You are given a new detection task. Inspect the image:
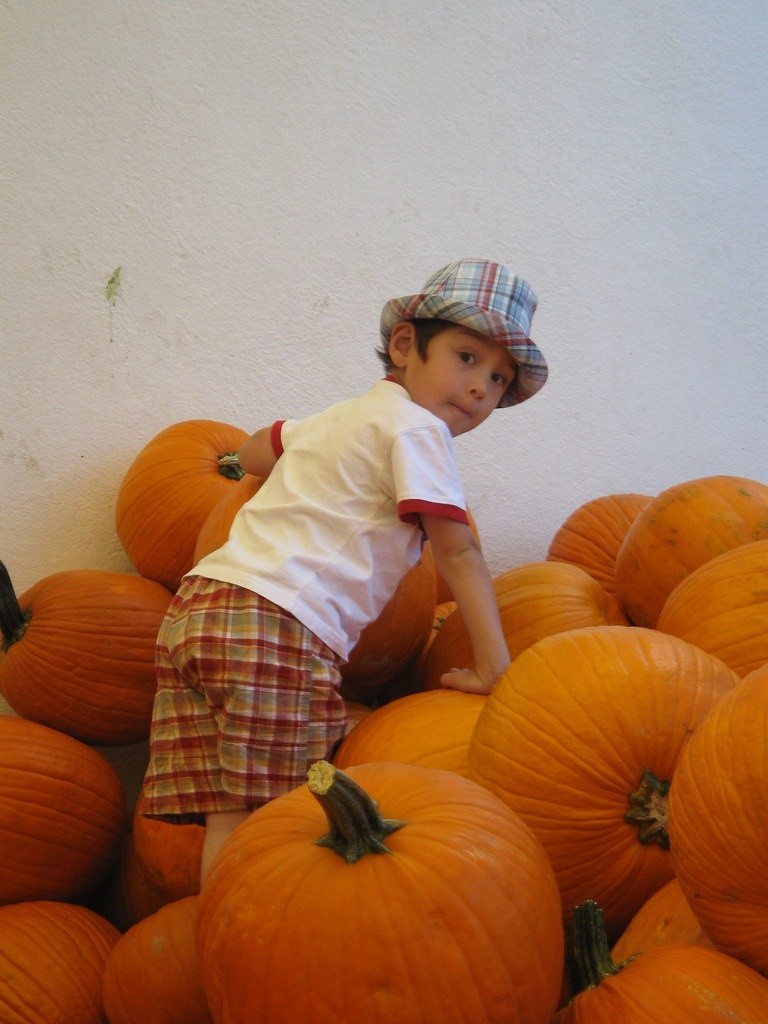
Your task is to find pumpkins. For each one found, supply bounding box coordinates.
[0,418,768,1024]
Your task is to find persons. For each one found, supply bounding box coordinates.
[140,258,549,893]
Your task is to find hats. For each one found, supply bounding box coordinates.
[380,257,548,410]
